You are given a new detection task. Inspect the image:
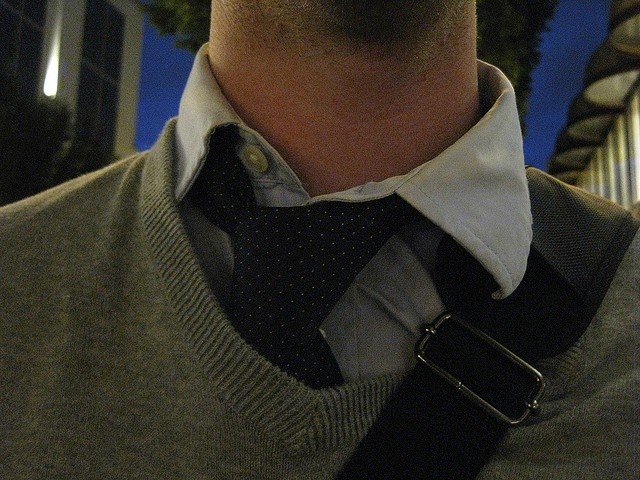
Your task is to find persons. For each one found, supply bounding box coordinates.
[0,0,640,480]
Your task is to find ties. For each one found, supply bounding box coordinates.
[187,138,418,389]
